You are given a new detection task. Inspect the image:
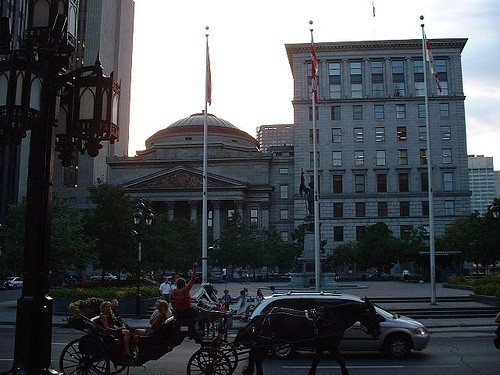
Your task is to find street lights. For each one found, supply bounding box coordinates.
[129,199,157,314]
[0,13,122,374]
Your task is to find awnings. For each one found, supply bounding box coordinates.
[419,251,462,255]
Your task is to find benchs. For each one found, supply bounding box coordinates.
[141,316,175,338]
[86,315,120,343]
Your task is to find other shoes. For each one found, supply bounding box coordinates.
[123,353,135,360]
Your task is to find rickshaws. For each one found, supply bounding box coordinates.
[60,296,240,375]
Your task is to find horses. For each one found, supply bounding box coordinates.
[233,295,382,375]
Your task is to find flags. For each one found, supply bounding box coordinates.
[425,38,442,93]
[311,42,319,103]
[207,41,211,104]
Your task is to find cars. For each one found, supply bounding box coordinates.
[89,272,117,281]
[244,291,431,357]
[2,276,23,289]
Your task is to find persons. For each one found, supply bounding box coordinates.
[299,168,308,197]
[255,288,264,302]
[236,286,252,302]
[308,181,313,215]
[130,299,168,351]
[270,286,278,294]
[214,289,231,311]
[238,265,250,284]
[159,275,180,303]
[100,297,133,356]
[403,267,410,284]
[170,262,206,336]
[375,263,382,281]
